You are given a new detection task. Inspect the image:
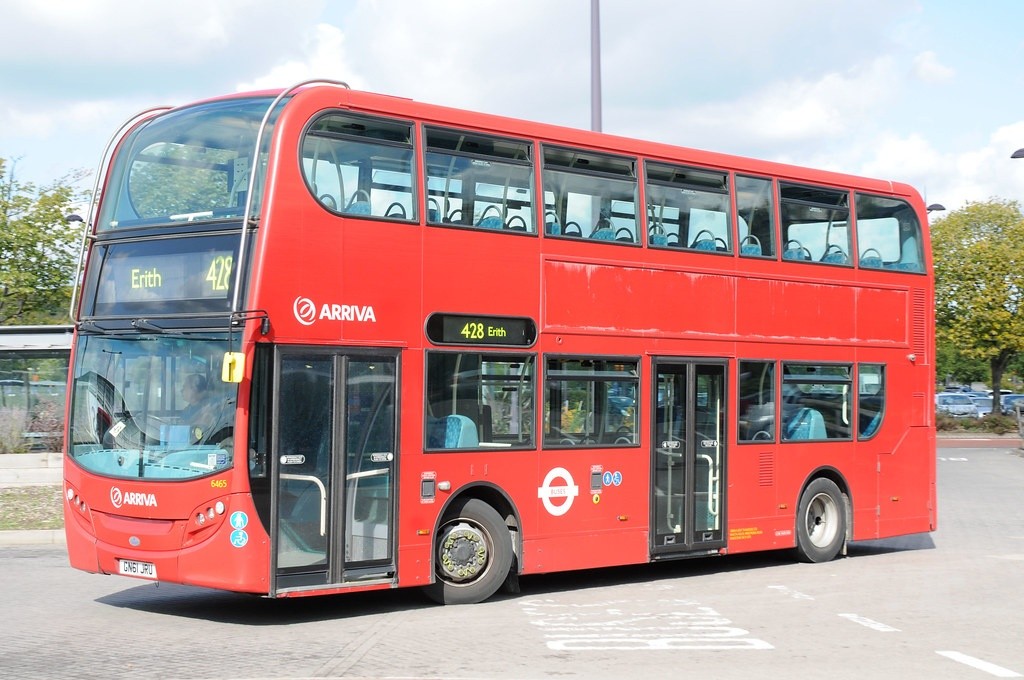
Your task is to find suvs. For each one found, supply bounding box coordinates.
[944,385,975,394]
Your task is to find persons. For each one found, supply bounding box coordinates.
[166,374,214,430]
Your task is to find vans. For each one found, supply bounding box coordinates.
[859,373,884,395]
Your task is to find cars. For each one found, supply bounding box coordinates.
[971,397,995,417]
[606,395,637,417]
[981,390,1012,397]
[999,394,1024,414]
[936,392,979,420]
[965,392,989,398]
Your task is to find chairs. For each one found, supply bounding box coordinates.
[448,209,468,225]
[819,244,845,264]
[689,229,716,251]
[860,247,883,268]
[429,197,442,222]
[343,187,373,215]
[474,205,503,228]
[546,211,561,235]
[506,215,527,231]
[741,235,762,255]
[835,250,848,260]
[563,221,582,237]
[383,201,407,218]
[716,237,728,251]
[649,224,669,246]
[616,227,634,241]
[588,217,616,240]
[783,238,805,260]
[357,378,883,522]
[319,192,338,209]
[799,246,812,260]
[667,231,682,246]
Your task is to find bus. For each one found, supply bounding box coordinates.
[72,79,941,602]
